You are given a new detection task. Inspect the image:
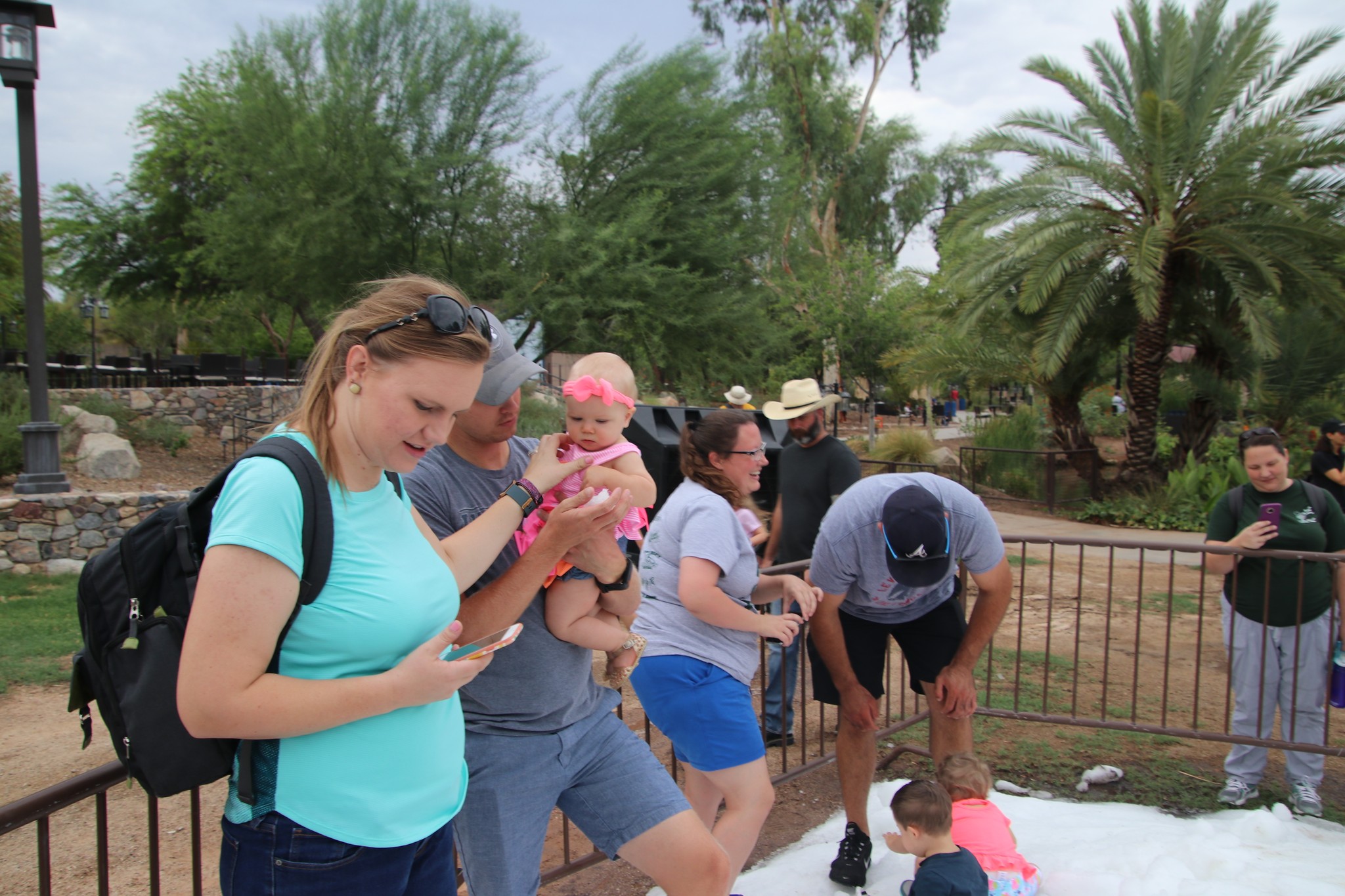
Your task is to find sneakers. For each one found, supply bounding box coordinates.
[1217,777,1259,805]
[1288,784,1323,817]
[829,821,872,888]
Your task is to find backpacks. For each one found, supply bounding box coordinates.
[66,435,335,799]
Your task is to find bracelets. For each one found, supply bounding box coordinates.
[516,478,544,505]
[595,557,632,593]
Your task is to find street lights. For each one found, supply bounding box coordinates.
[820,380,851,436]
[80,290,111,385]
[0,0,70,496]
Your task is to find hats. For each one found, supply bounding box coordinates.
[723,385,753,405]
[881,484,951,589]
[762,378,843,421]
[460,305,550,406]
[1321,418,1345,435]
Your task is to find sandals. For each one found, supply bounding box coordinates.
[603,632,647,691]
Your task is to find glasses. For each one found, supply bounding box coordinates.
[363,294,493,346]
[718,442,767,461]
[1238,426,1280,442]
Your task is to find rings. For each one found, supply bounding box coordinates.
[529,449,537,458]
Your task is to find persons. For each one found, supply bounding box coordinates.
[1309,420,1344,513]
[804,471,1016,887]
[838,385,959,426]
[881,747,1043,895]
[1202,427,1345,816]
[888,778,989,896]
[173,275,597,895]
[752,376,862,746]
[732,490,770,549]
[1112,390,1127,416]
[719,385,756,409]
[388,308,738,895]
[513,353,657,688]
[625,410,823,879]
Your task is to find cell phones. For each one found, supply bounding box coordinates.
[766,612,803,644]
[439,623,524,660]
[1260,503,1281,539]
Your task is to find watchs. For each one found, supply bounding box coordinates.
[500,480,537,517]
